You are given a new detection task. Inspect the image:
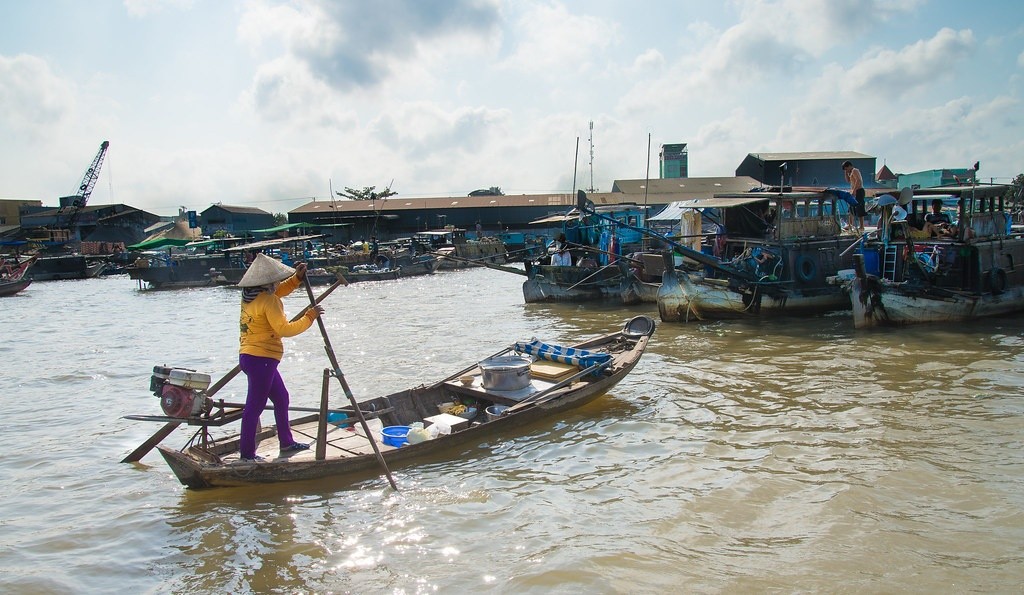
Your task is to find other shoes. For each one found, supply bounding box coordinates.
[280,442,309,451]
[242,455,266,461]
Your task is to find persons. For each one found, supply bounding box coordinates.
[842,160,865,231]
[246,249,261,263]
[924,199,950,228]
[411,237,420,251]
[370,237,378,254]
[783,201,793,218]
[476,222,481,239]
[306,240,312,251]
[428,234,441,243]
[238,253,325,461]
[361,239,368,253]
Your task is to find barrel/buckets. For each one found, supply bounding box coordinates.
[381,425,413,448]
[853,239,881,277]
[438,402,454,413]
[485,405,511,422]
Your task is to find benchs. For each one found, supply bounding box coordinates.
[445,375,557,406]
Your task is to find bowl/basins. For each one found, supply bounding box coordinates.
[459,375,475,386]
[459,408,478,419]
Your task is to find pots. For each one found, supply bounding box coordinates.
[478,355,531,391]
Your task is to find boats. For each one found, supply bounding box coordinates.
[0,131,1024,334]
[123,311,658,492]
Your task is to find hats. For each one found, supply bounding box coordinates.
[238,253,296,287]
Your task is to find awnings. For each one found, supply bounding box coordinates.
[646,200,705,221]
[679,197,770,208]
[528,215,586,224]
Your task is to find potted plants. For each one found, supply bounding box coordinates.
[214,229,227,239]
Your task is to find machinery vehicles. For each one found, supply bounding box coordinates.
[20,139,110,257]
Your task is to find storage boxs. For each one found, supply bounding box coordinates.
[530,359,581,383]
[423,412,469,438]
[459,407,477,419]
[963,211,1012,238]
[169,370,212,390]
[137,259,150,269]
[152,365,172,379]
[898,168,976,189]
[838,268,857,281]
[353,418,384,443]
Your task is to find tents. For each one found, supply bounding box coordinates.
[264,221,356,237]
[127,238,222,256]
